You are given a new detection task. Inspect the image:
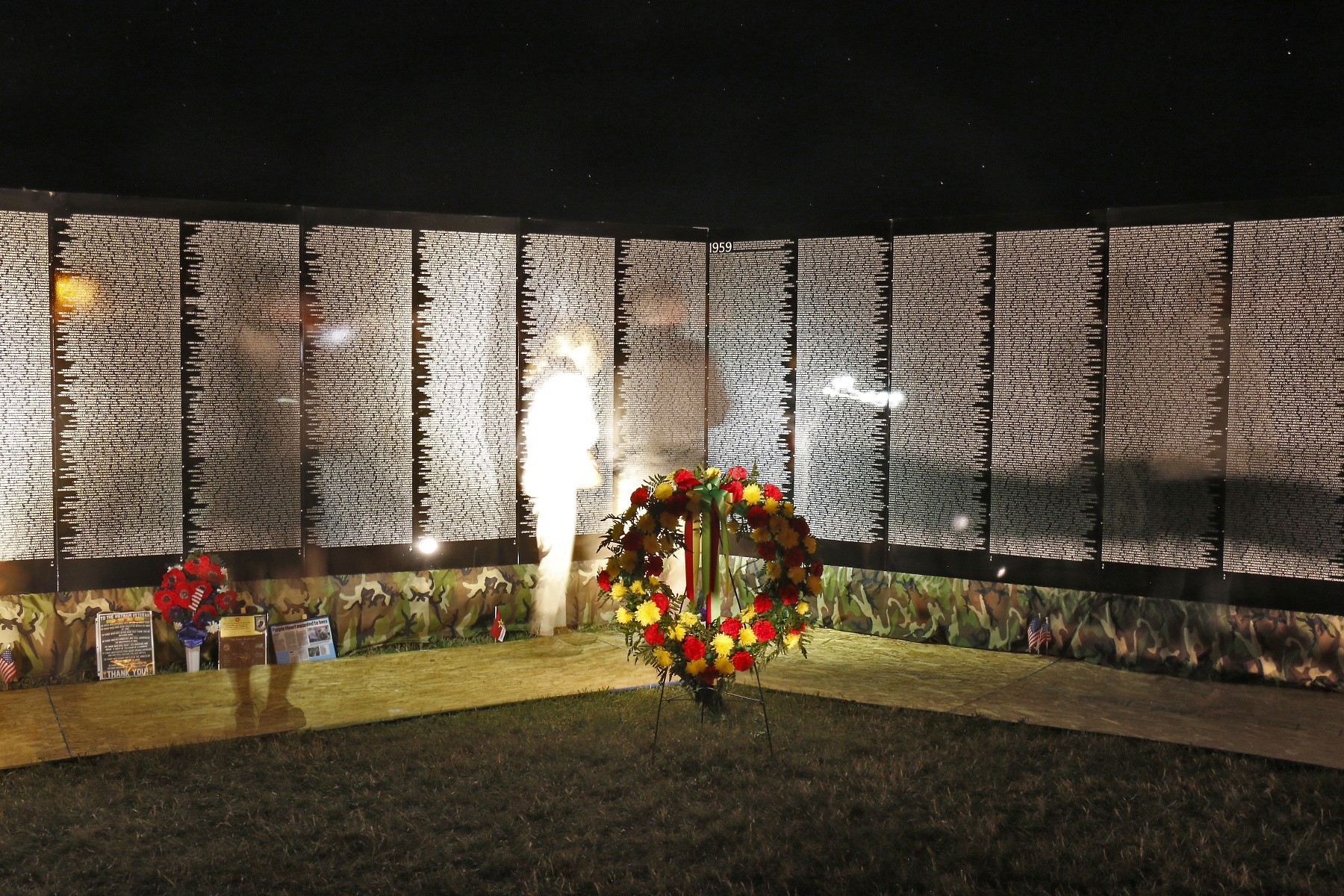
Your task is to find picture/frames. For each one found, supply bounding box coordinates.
[267,614,339,663]
[215,613,270,669]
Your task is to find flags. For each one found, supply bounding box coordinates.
[1027,616,1052,653]
[489,609,506,642]
[0,647,18,686]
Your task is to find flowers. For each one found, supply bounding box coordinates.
[593,465,824,692]
[153,549,238,637]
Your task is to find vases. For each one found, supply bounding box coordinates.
[181,638,202,673]
